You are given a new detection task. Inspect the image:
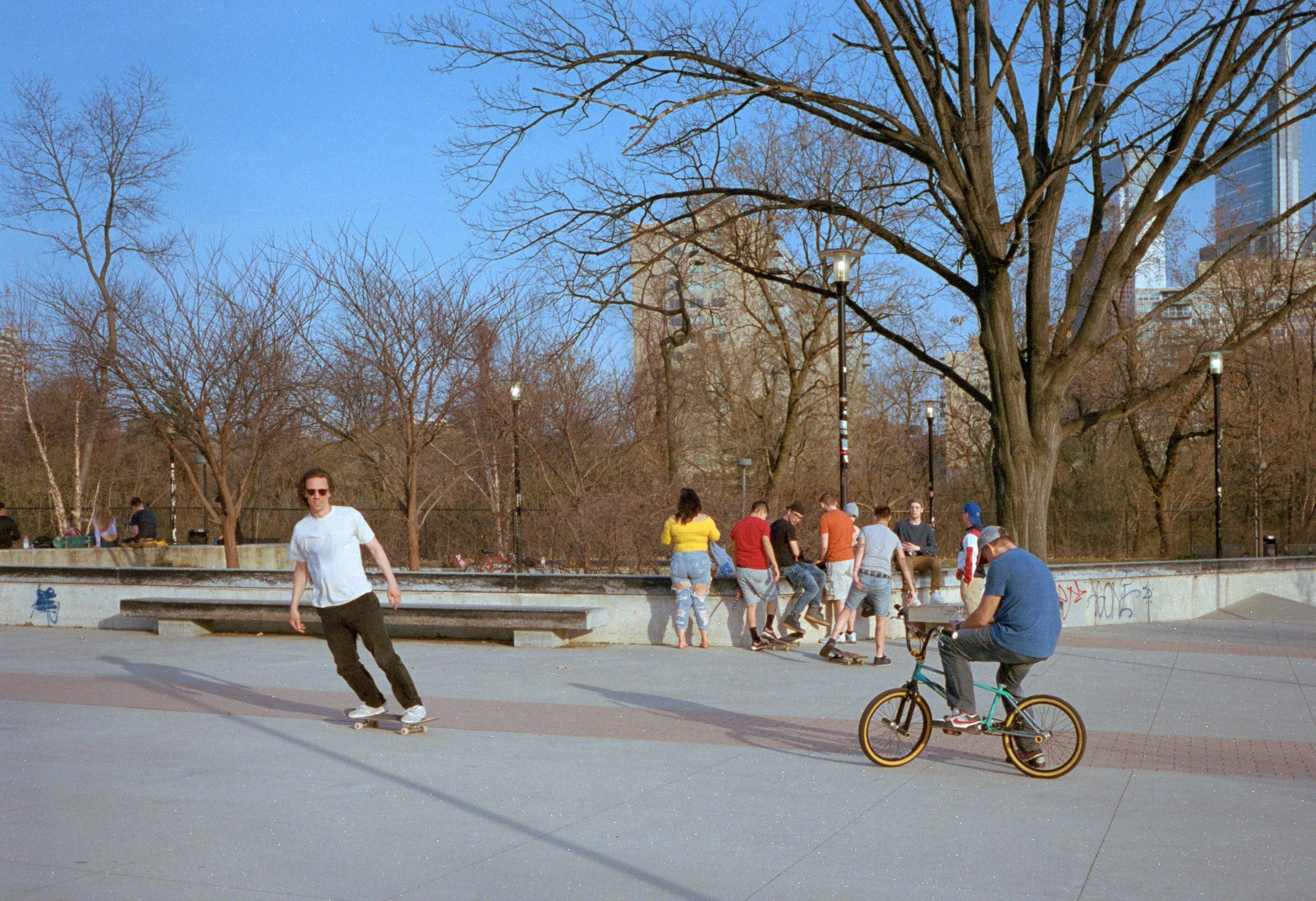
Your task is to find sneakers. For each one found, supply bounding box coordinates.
[844,632,857,643]
[907,590,921,607]
[929,589,948,605]
[805,606,830,626]
[782,615,806,634]
[1005,748,1046,768]
[873,653,892,666]
[400,705,426,723]
[760,627,778,641]
[819,638,836,658]
[347,700,389,719]
[819,632,846,643]
[860,605,876,617]
[929,710,982,735]
[750,636,768,650]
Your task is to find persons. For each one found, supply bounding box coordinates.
[770,501,829,635]
[0,502,20,549]
[956,502,986,616]
[938,526,1062,769]
[661,489,720,648]
[93,503,120,548]
[121,497,157,543]
[893,500,948,607]
[288,468,426,724]
[819,505,915,666]
[812,488,859,643]
[843,502,860,536]
[730,501,779,652]
[214,496,242,545]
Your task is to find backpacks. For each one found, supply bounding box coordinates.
[65,526,83,536]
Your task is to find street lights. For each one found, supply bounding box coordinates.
[158,414,182,545]
[1200,349,1235,560]
[917,400,943,531]
[818,247,867,516]
[737,457,752,519]
[502,379,530,574]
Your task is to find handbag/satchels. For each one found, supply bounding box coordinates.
[710,541,736,578]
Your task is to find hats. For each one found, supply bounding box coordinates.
[963,502,982,525]
[842,502,859,519]
[786,501,804,516]
[910,498,926,514]
[976,526,1009,566]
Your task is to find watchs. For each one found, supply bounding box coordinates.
[956,623,961,630]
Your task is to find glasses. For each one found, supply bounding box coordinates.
[790,510,803,521]
[304,489,329,497]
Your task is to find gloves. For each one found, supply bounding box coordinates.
[214,536,219,545]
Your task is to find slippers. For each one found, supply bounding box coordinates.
[699,643,710,648]
[678,644,688,649]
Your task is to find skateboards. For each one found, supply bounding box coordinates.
[344,708,440,735]
[827,647,868,665]
[53,536,95,548]
[769,633,803,651]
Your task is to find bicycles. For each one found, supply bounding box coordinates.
[462,548,531,575]
[858,603,1088,779]
